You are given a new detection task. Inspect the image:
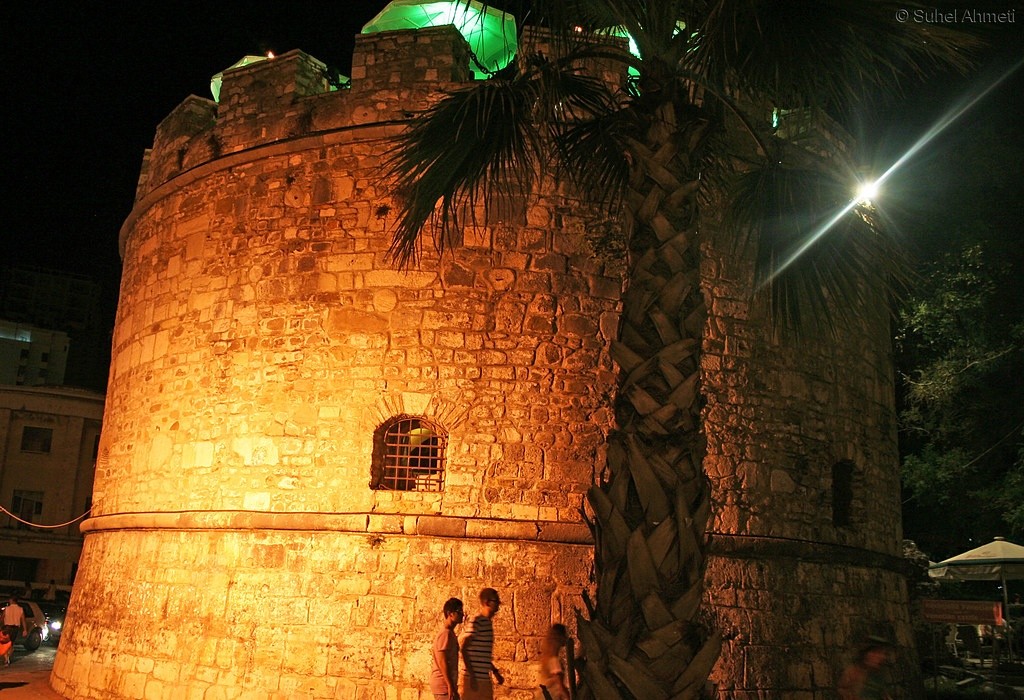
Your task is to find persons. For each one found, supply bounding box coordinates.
[540,623,570,700]
[992,618,1013,638]
[19,583,32,599]
[0,597,28,667]
[459,589,504,700]
[43,579,56,600]
[430,598,461,700]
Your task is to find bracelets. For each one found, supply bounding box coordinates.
[493,669,498,674]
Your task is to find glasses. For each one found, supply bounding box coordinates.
[489,599,500,604]
[451,610,463,614]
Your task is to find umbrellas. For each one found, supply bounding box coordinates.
[211,53,349,102]
[928,536,1024,663]
[361,0,520,80]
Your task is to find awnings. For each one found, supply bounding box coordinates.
[0,540,82,562]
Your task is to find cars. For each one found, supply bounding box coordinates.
[0,599,66,651]
